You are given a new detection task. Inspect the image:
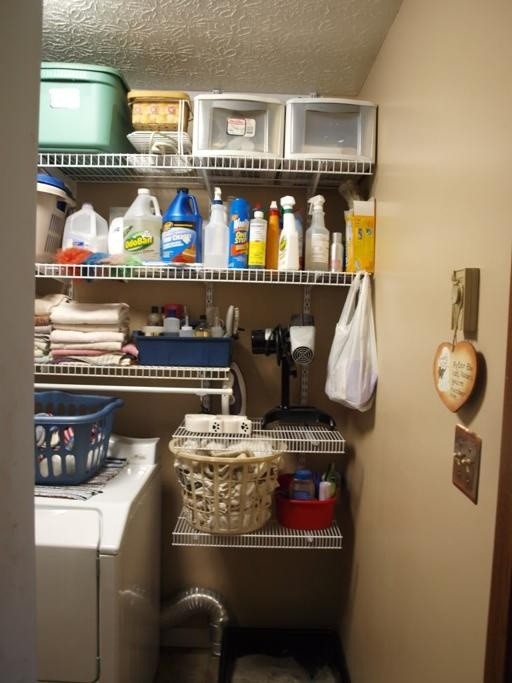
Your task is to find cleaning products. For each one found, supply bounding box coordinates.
[59,185,346,273]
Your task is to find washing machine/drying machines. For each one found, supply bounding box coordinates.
[32,432,170,683]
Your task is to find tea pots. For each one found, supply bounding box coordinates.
[211,307,225,337]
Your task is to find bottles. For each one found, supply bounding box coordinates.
[194,315,211,337]
[163,308,180,336]
[180,313,194,336]
[320,460,342,488]
[330,231,344,272]
[229,196,250,268]
[147,305,164,326]
[289,469,316,500]
[249,202,268,268]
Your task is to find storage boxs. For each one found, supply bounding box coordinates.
[38,62,142,181]
[192,94,285,185]
[132,331,232,367]
[277,98,377,189]
[36,173,77,264]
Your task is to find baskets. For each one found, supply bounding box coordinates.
[34,391,124,486]
[169,432,287,535]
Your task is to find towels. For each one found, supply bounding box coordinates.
[34,294,139,367]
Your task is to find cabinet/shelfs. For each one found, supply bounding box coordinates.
[34,153,374,402]
[171,417,348,549]
